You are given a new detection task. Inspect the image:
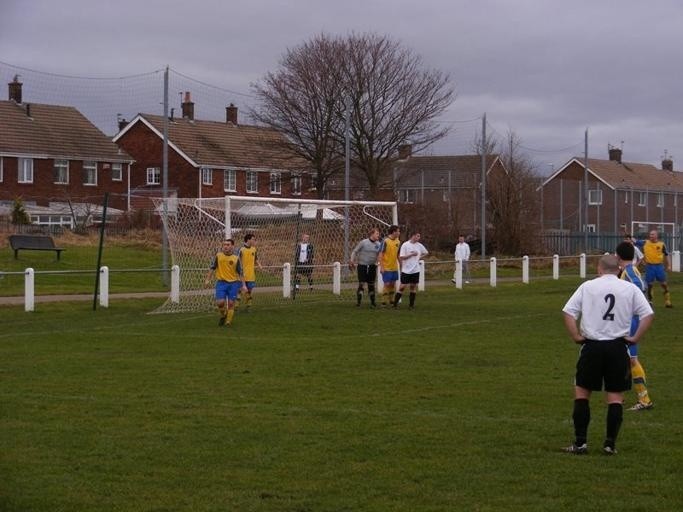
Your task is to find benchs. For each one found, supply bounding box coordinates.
[8,234,67,261]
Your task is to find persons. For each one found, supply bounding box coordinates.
[347,227,381,309]
[379,223,401,307]
[616,233,644,280]
[203,239,248,327]
[292,233,315,295]
[392,229,429,309]
[634,228,672,309]
[450,234,473,284]
[607,241,655,414]
[234,234,263,308]
[558,253,655,456]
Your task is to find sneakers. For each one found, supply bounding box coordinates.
[218,316,226,326]
[604,446,617,454]
[562,442,588,453]
[627,401,653,410]
